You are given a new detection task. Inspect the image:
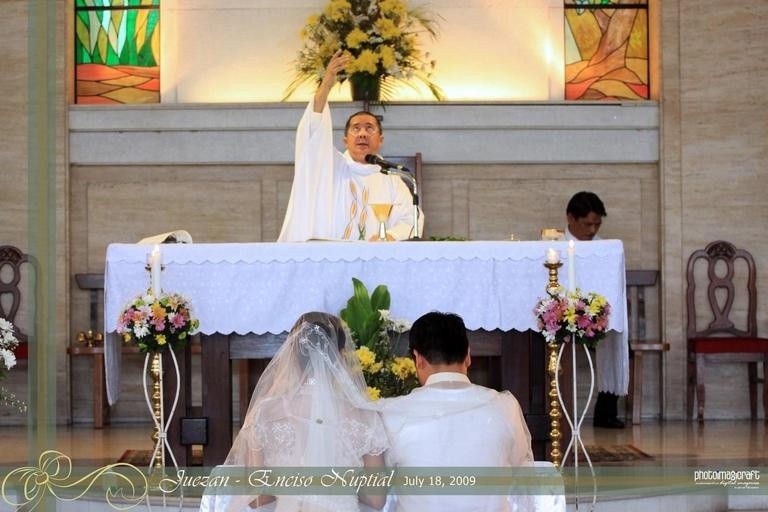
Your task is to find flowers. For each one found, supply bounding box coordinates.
[114,287,201,355]
[279,0,448,112]
[531,288,612,348]
[340,276,422,400]
[0,317,28,416]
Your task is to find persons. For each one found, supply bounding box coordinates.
[272,49,428,244]
[198,310,392,511]
[556,189,629,430]
[378,311,538,511]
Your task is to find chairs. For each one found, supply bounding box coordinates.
[0,244,44,431]
[683,239,768,424]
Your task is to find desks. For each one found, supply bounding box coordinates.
[103,238,628,461]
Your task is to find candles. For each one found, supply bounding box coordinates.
[547,251,560,264]
[567,246,576,295]
[150,249,162,297]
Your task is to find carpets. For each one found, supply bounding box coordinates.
[115,442,658,467]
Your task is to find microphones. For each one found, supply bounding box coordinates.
[365,154,410,172]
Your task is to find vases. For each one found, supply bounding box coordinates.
[348,76,382,104]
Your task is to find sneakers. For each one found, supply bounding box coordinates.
[593,416,626,428]
